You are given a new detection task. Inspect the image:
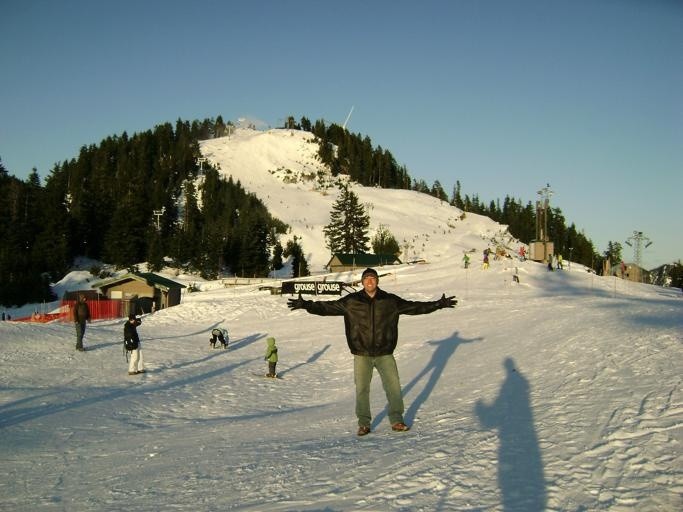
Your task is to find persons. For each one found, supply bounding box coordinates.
[495,245,501,258]
[1,311,5,321]
[6,314,10,321]
[264,336,279,378]
[461,254,469,269]
[123,313,148,375]
[73,294,91,351]
[556,252,563,270]
[546,253,553,272]
[482,247,495,257]
[286,268,458,436]
[209,328,229,349]
[511,267,519,283]
[482,254,489,270]
[519,246,527,261]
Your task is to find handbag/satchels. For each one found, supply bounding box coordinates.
[123,336,138,351]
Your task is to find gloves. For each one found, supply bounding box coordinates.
[438,292,458,309]
[286,292,308,312]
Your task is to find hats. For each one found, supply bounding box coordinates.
[361,267,378,280]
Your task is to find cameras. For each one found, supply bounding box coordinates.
[138,320,141,324]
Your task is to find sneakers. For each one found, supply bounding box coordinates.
[357,427,371,436]
[391,422,409,432]
[128,370,145,375]
[266,373,279,378]
[76,346,86,351]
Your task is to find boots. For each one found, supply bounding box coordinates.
[210,342,227,350]
